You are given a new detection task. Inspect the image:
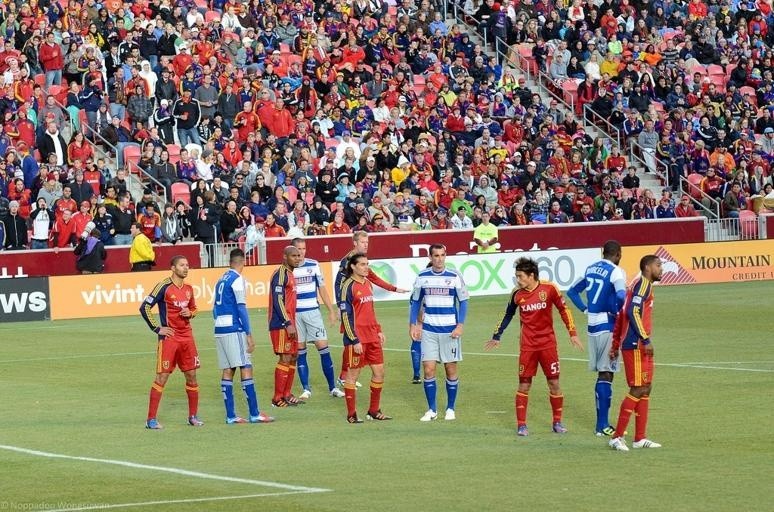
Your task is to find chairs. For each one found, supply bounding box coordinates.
[0,0,772,249]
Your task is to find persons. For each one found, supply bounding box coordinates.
[434,1,774,238]
[0,1,701,274]
[290,237,346,399]
[139,255,205,430]
[608,255,663,452]
[334,230,412,390]
[482,257,584,436]
[339,252,393,423]
[566,240,629,436]
[409,261,433,384]
[409,244,469,421]
[212,249,275,424]
[268,245,306,408]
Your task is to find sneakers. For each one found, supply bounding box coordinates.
[596,425,628,436]
[366,410,392,420]
[632,438,662,449]
[413,376,421,383]
[271,391,311,407]
[420,409,437,421]
[146,420,161,429]
[225,411,275,424]
[552,423,567,433]
[346,412,363,423]
[330,377,361,397]
[517,426,528,435]
[187,417,204,427]
[445,408,455,420]
[609,438,630,452]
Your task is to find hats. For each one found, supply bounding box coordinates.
[630,108,636,113]
[503,152,537,170]
[366,155,376,163]
[495,136,503,141]
[417,133,429,142]
[550,100,557,105]
[764,127,773,134]
[9,201,19,210]
